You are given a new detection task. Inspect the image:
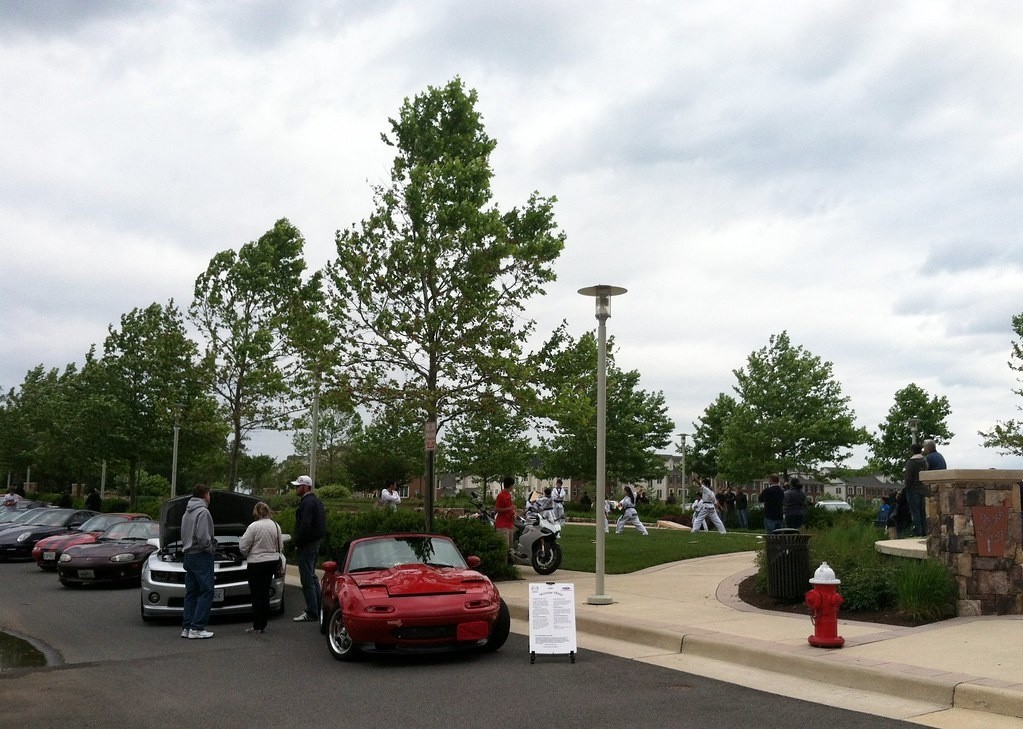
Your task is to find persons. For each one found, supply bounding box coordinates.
[580,491,592,507]
[472,508,495,526]
[84,488,103,512]
[459,509,471,518]
[758,473,806,534]
[690,475,726,533]
[526,478,566,539]
[15,480,25,498]
[291,475,326,622]
[434,508,453,520]
[382,479,401,512]
[239,502,284,634]
[3,486,18,507]
[716,486,749,528]
[604,493,611,532]
[494,476,517,566]
[59,488,73,508]
[281,489,287,495]
[181,483,218,638]
[877,439,946,539]
[373,492,376,498]
[616,486,649,536]
[667,492,677,504]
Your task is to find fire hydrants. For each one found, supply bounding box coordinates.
[804,561,846,649]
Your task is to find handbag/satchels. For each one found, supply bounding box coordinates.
[277,566,285,577]
[377,499,390,508]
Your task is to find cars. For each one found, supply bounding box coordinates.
[31,511,161,573]
[814,500,854,513]
[137,487,292,624]
[55,520,161,589]
[0,492,105,560]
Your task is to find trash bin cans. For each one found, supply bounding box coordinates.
[762,529,813,607]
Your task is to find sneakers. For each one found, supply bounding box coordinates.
[181,628,189,638]
[188,629,214,639]
[293,612,316,621]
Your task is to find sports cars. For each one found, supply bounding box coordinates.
[317,531,512,663]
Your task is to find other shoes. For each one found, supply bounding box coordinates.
[246,627,262,633]
[906,534,922,538]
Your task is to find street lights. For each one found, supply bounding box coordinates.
[676,433,691,514]
[576,284,629,606]
[170,402,189,498]
[301,365,328,494]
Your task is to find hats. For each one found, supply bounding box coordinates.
[291,476,312,486]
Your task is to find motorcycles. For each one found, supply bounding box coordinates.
[467,489,562,575]
